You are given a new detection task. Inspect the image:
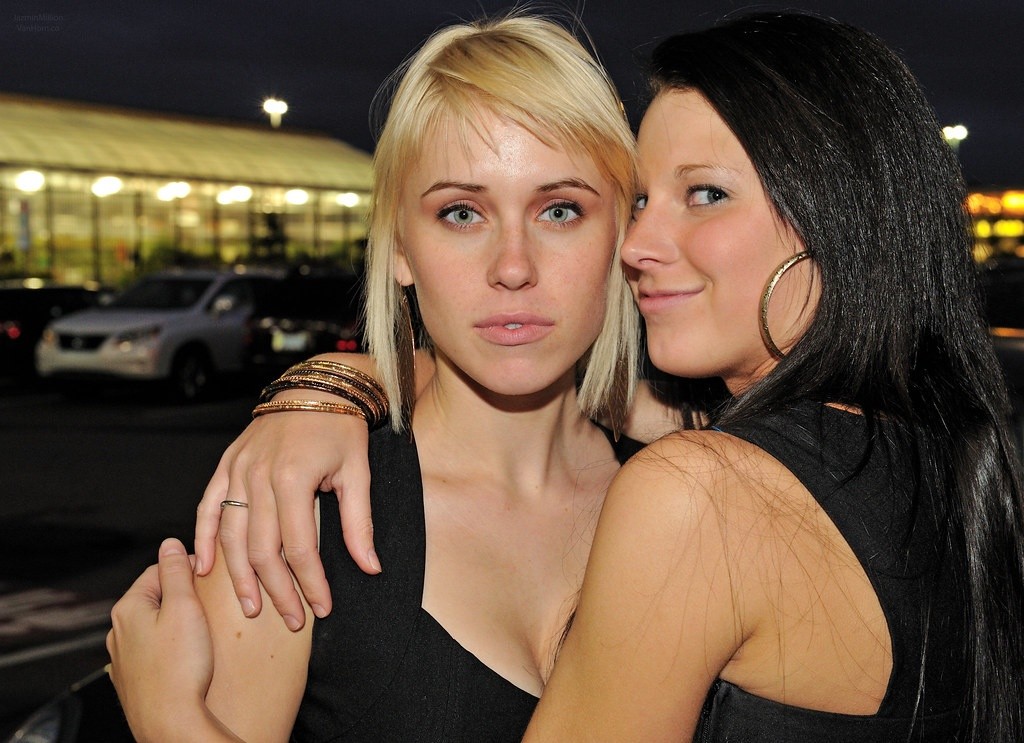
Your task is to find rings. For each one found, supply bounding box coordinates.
[220,499,250,511]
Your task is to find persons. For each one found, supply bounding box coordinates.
[75,8,726,743]
[104,3,1024,743]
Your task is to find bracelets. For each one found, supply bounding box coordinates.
[251,397,363,427]
[265,358,392,424]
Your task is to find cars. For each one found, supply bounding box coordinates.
[244,261,383,398]
[0,282,109,381]
[34,265,280,404]
